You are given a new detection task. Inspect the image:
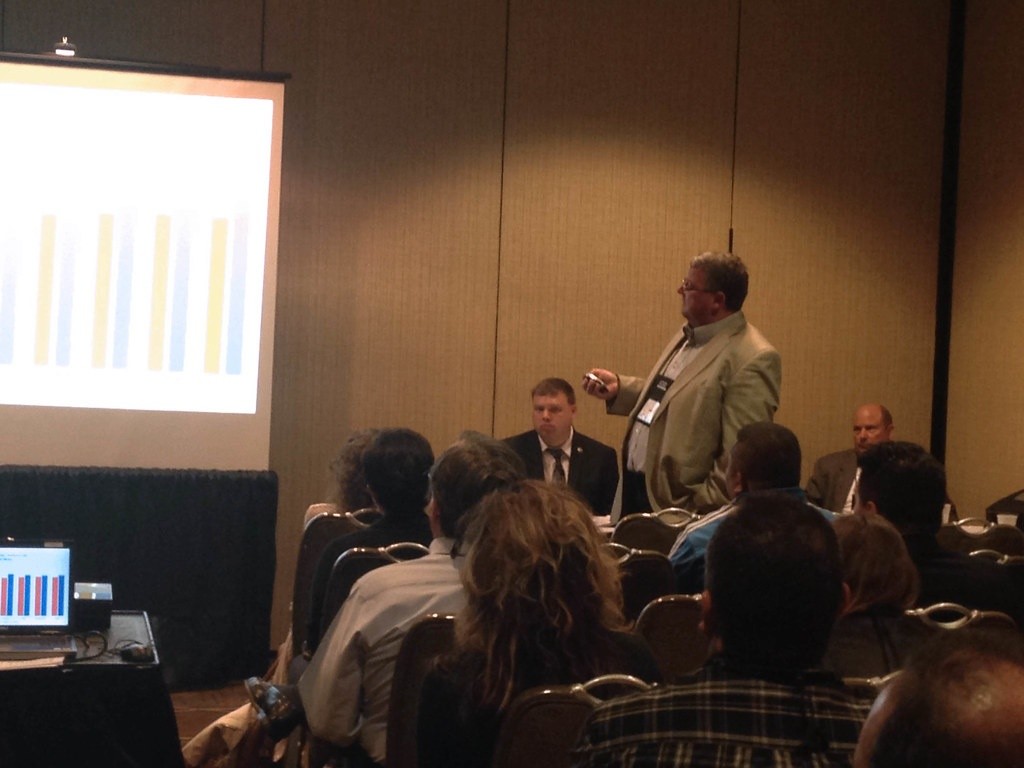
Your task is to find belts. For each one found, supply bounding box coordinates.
[622,471,646,486]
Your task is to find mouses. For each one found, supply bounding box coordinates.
[120,642,152,662]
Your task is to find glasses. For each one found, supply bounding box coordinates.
[681,278,716,296]
[328,455,340,470]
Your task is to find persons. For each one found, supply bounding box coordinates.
[415,478,665,767]
[304,429,435,653]
[833,512,939,677]
[668,420,838,595]
[853,627,1024,768]
[502,378,620,515]
[305,428,380,526]
[806,404,895,513]
[580,250,783,521]
[852,441,1024,623]
[296,428,528,768]
[565,495,871,768]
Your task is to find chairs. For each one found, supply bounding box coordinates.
[294,508,1024,768]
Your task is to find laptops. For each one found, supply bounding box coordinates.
[0,539,77,660]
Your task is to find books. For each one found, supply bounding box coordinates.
[0,635,77,661]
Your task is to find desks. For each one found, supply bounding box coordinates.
[0,608,187,768]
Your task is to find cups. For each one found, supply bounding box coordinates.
[996,514,1018,527]
[942,504,952,524]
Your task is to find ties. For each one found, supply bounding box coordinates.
[546,449,567,489]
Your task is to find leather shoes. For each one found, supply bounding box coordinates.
[244,676,305,743]
